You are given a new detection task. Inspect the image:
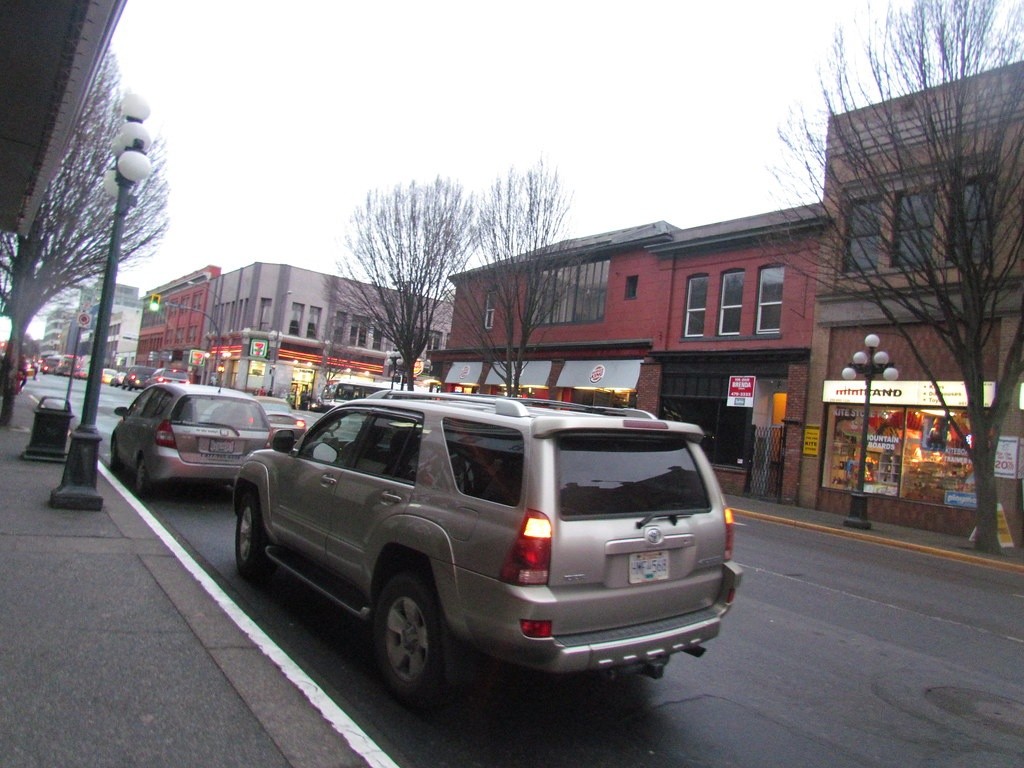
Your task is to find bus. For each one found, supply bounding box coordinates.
[320,378,409,412]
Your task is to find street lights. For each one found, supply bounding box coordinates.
[47,98,152,511]
[838,332,900,527]
[388,352,401,393]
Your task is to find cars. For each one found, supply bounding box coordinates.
[122,366,155,391]
[101,368,118,384]
[144,368,190,391]
[251,394,309,447]
[109,371,128,388]
[38,350,92,381]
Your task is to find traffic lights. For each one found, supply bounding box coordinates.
[149,294,160,312]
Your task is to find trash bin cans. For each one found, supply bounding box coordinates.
[22,396,75,464]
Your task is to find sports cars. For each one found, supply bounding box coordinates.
[106,382,279,499]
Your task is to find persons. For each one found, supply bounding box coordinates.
[32,354,39,380]
[343,390,349,399]
[21,353,27,393]
[208,380,214,386]
[252,388,258,396]
[299,390,312,411]
[267,388,273,396]
[259,386,266,396]
[215,378,223,387]
[289,388,297,409]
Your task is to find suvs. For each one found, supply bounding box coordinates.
[231,389,745,712]
